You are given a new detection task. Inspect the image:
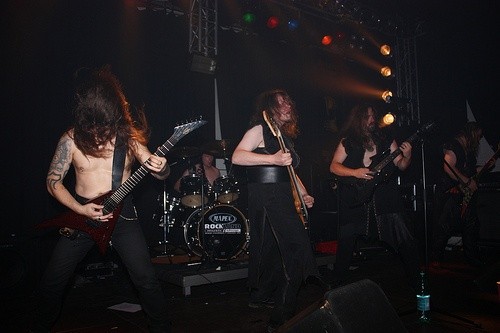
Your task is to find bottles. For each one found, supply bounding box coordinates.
[416,272,432,322]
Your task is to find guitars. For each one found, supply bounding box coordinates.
[331,122,436,209]
[262,110,308,226]
[443,143,500,217]
[30,116,209,257]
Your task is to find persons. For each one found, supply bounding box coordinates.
[175,148,220,203]
[435,122,496,245]
[330,105,428,293]
[42,65,172,333]
[230,89,320,332]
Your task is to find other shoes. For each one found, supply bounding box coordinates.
[29,305,60,333]
[247,294,277,309]
[267,322,279,333]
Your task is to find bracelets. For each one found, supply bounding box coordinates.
[404,156,412,160]
[302,194,309,197]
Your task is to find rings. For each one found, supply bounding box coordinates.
[160,161,162,165]
[98,217,101,220]
[365,174,368,177]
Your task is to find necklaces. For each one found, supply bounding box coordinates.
[368,145,374,152]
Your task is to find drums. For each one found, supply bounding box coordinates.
[213,175,241,204]
[183,205,249,261]
[180,175,208,207]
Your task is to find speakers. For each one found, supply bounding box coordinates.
[276,279,406,333]
[452,181,500,251]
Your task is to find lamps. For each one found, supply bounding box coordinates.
[188,51,219,77]
[374,39,399,124]
